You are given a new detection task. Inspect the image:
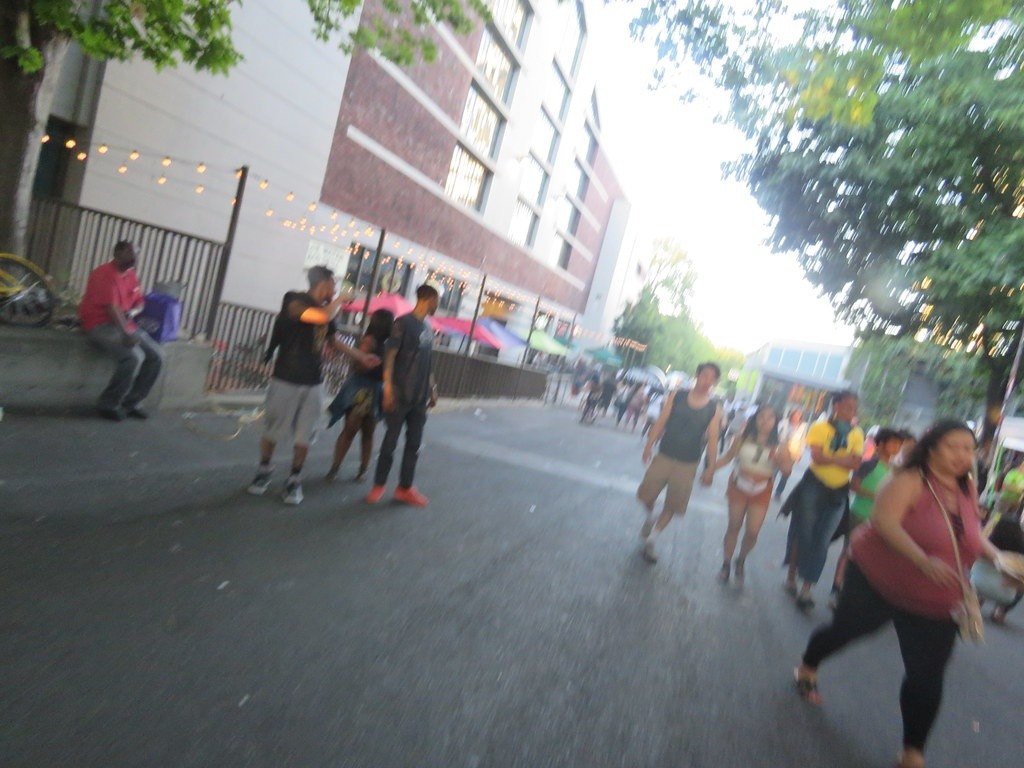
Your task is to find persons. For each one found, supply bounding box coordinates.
[793,417,1024,768]
[367,285,437,507]
[323,308,394,483]
[636,361,720,564]
[783,390,864,610]
[969,493,1023,628]
[716,406,792,587]
[246,266,381,505]
[831,427,904,610]
[79,241,162,421]
[577,363,1023,540]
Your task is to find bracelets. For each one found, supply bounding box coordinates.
[430,384,437,393]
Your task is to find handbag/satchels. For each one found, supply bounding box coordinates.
[614,386,626,408]
[732,470,769,497]
[135,291,181,342]
[949,583,985,647]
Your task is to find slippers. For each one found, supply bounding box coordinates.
[792,667,822,706]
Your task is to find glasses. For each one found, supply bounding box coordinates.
[752,444,763,464]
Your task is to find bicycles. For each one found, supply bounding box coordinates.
[0,254,59,329]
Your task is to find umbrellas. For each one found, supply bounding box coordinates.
[341,291,624,372]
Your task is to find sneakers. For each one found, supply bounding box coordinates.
[283,473,304,503]
[394,485,429,506]
[248,465,274,495]
[367,485,386,501]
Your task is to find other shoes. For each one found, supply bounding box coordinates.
[96,405,121,421]
[129,409,149,419]
[326,472,338,480]
[735,560,745,581]
[641,514,656,537]
[356,472,367,482]
[793,589,815,608]
[716,564,730,583]
[643,541,657,564]
[786,582,798,598]
